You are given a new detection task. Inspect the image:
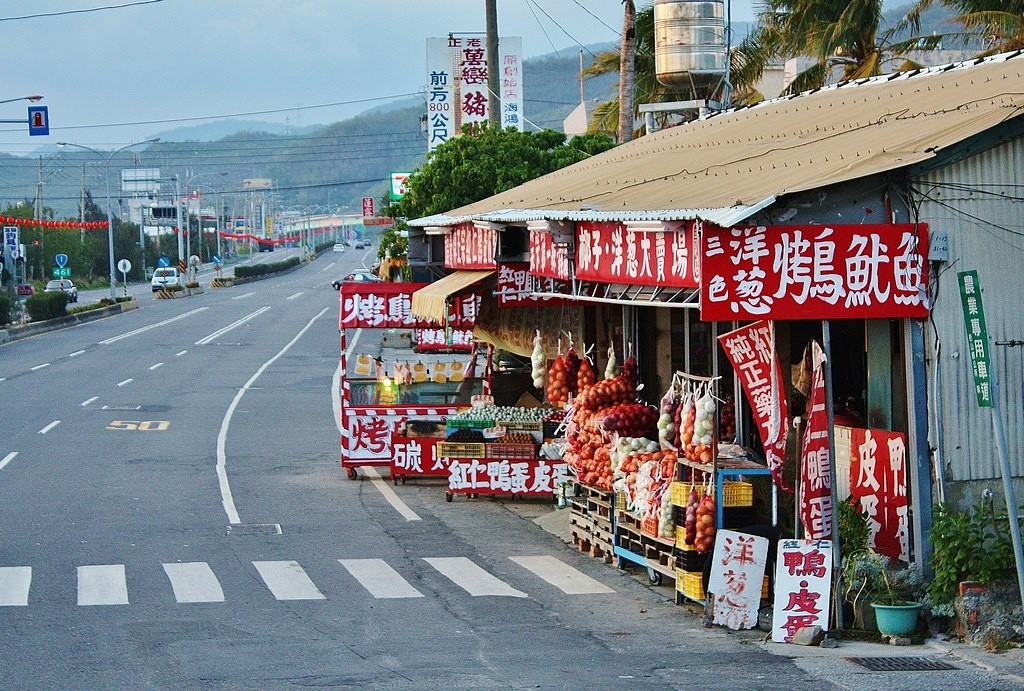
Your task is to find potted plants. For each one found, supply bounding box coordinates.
[850,554,914,631]
[851,548,923,637]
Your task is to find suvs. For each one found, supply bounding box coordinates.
[44,279,78,303]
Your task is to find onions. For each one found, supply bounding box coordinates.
[531,344,737,554]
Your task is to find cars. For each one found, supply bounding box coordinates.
[17,284,35,295]
[363,239,372,246]
[332,272,383,290]
[355,242,364,249]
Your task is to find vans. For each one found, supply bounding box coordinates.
[151,267,180,291]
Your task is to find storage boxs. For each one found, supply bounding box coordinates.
[676,523,738,552]
[675,505,748,530]
[447,419,494,428]
[675,567,769,602]
[495,420,542,430]
[611,491,627,510]
[485,442,534,459]
[674,547,711,571]
[671,479,753,508]
[640,513,659,538]
[436,442,485,459]
[547,422,566,437]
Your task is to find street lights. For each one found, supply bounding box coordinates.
[55,137,161,301]
[154,172,228,274]
[189,181,348,267]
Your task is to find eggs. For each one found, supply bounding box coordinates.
[445,404,554,445]
